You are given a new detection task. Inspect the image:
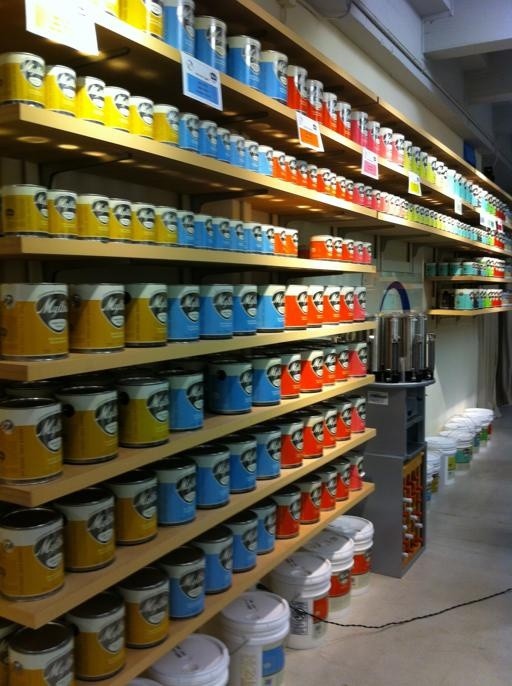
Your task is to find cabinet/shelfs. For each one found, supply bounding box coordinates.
[0,0,512,686]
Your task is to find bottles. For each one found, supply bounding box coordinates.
[2,0,512,686]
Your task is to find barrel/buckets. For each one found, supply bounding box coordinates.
[424,407,494,516]
